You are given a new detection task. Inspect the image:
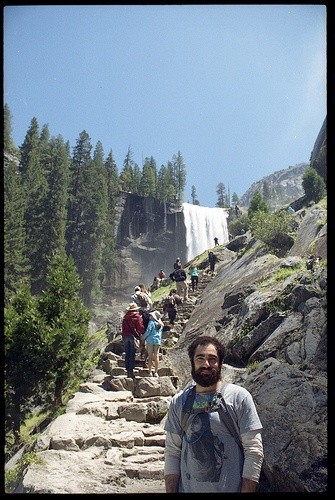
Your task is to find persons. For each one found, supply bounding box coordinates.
[189,264,199,292]
[214,237,219,246]
[169,265,188,301]
[131,283,153,325]
[174,257,182,269]
[153,269,166,289]
[164,336,264,493]
[122,303,145,379]
[165,288,183,324]
[140,311,164,377]
[209,252,216,271]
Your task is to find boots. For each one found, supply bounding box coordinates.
[127,369,135,378]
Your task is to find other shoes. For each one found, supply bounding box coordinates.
[154,373,158,377]
[149,373,153,377]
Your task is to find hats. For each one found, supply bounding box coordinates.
[149,311,164,327]
[126,303,140,310]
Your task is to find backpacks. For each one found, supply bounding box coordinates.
[134,292,149,308]
[174,268,185,281]
[165,294,176,308]
